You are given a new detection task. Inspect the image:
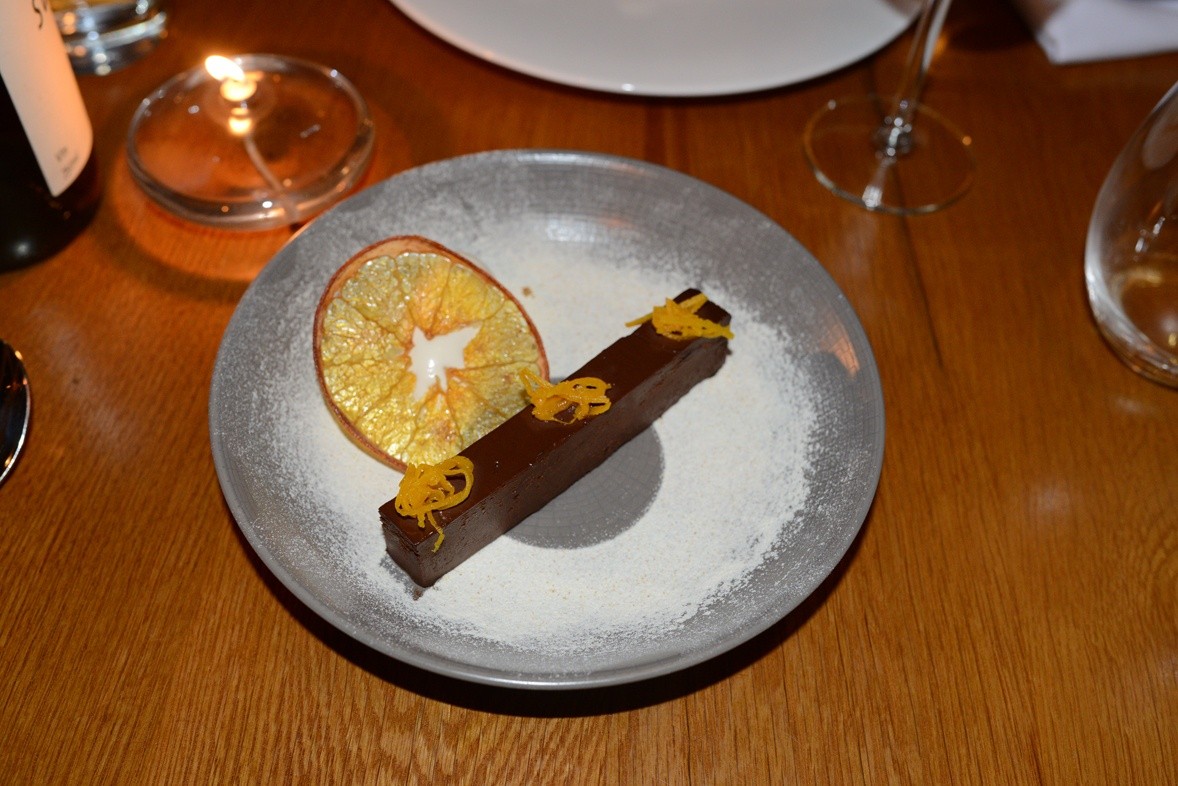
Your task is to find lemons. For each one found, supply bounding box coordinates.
[315,233,550,478]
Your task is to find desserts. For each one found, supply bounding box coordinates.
[378,287,732,586]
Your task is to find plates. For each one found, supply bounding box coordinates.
[210,144,889,699]
[383,0,929,96]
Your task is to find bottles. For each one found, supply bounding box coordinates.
[1084,58,1177,390]
[60,4,162,72]
